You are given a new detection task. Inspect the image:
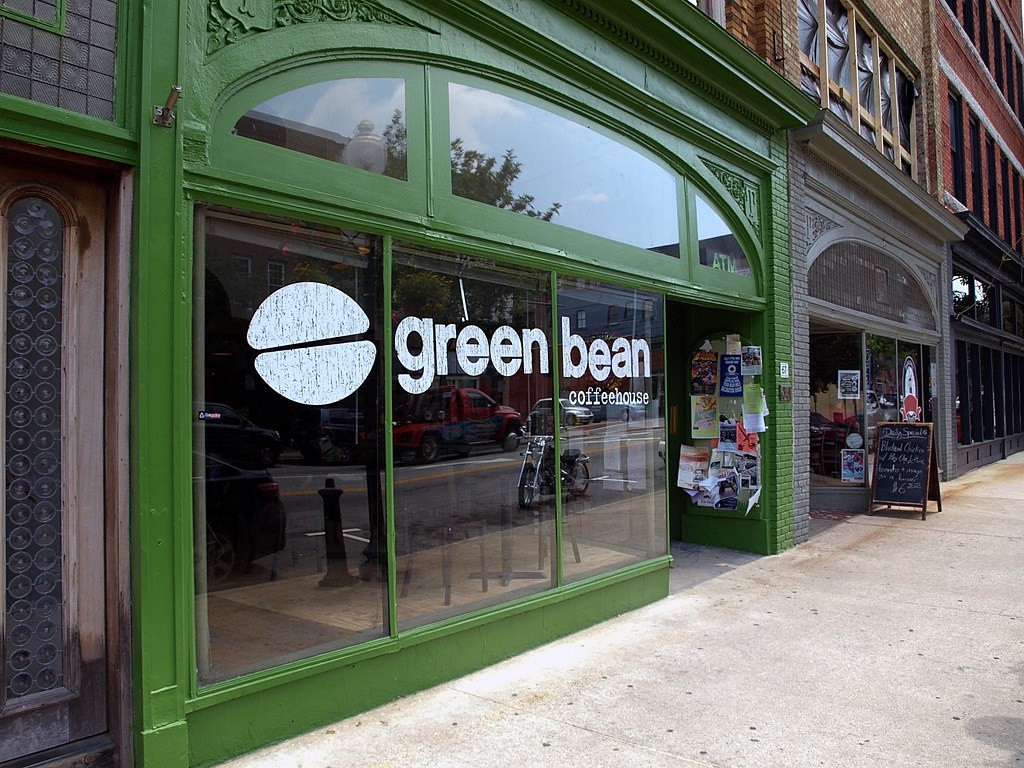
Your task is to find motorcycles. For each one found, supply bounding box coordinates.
[516,426,591,510]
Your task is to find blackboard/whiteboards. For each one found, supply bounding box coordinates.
[869,422,942,507]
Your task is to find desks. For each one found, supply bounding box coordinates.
[278,434,663,591]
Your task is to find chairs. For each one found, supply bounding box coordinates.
[399,461,581,606]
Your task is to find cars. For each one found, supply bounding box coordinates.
[589,394,648,423]
[190,448,287,587]
[866,390,898,438]
[377,384,525,466]
[299,404,371,463]
[205,403,284,470]
[533,397,594,426]
